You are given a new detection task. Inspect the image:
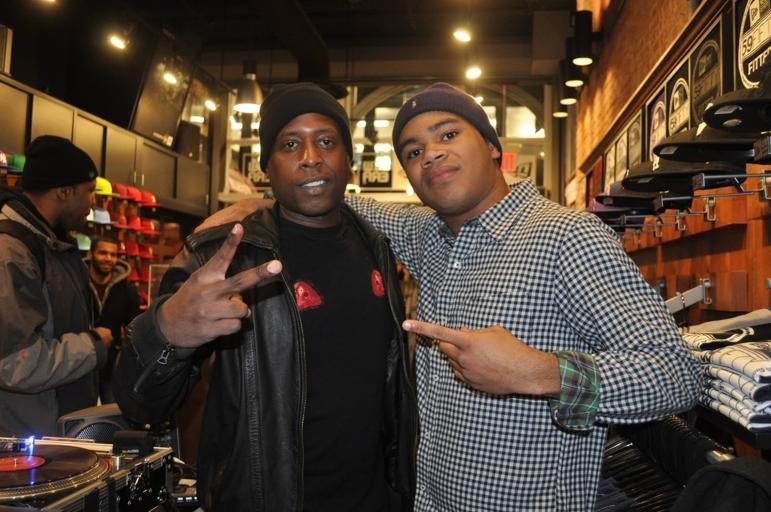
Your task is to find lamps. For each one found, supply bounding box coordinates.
[551,9,605,120]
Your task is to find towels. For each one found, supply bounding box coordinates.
[679,326,770,435]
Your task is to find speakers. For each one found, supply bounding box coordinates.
[56,402,183,460]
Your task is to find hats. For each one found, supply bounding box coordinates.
[0,151,16,169]
[23,134,98,190]
[75,176,161,303]
[8,155,26,172]
[392,83,502,166]
[260,83,352,172]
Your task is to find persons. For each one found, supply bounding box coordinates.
[1,132,116,443]
[111,79,424,511]
[83,235,144,405]
[191,78,705,509]
